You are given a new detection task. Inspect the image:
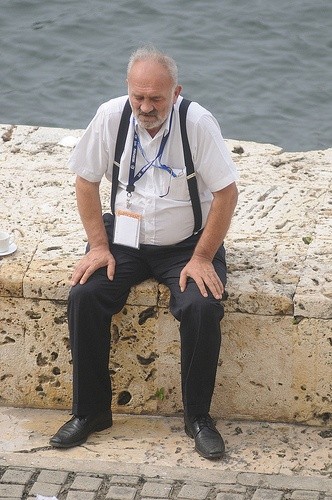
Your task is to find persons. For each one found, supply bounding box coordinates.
[49,49,238,461]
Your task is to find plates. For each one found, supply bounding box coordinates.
[0,243,17,255]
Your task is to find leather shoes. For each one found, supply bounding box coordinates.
[185,413,225,459]
[49,401,112,448]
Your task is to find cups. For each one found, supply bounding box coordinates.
[0,232,16,252]
